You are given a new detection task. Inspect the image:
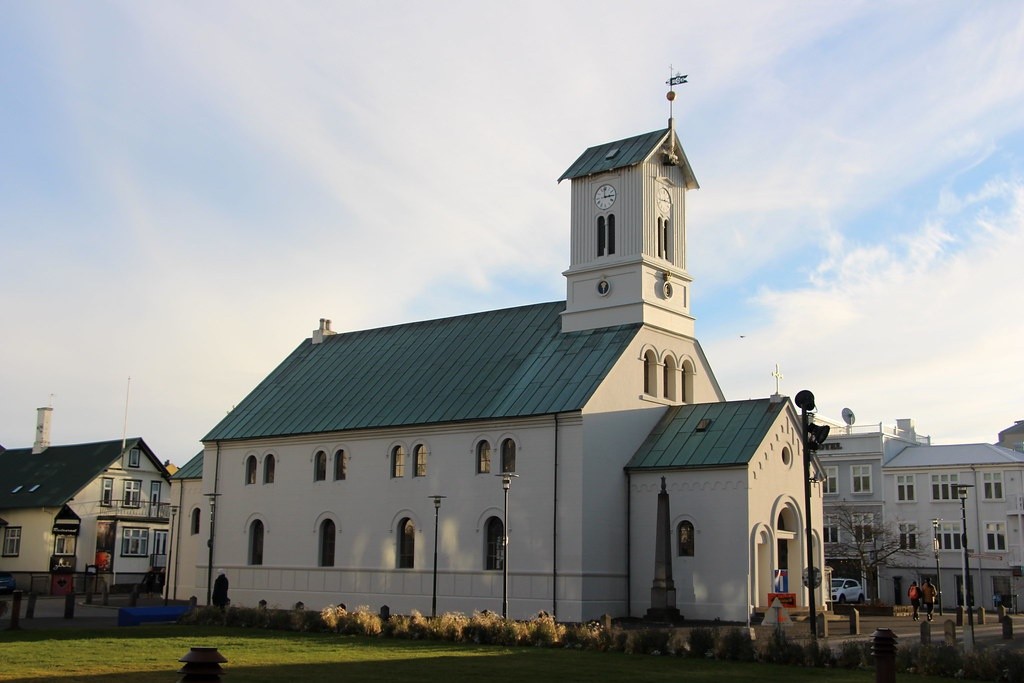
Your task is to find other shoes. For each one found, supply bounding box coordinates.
[917,618,920,620]
[913,617,917,621]
[927,614,933,622]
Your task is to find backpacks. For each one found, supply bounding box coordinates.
[909,586,919,599]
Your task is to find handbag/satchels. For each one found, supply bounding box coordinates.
[225,598,230,605]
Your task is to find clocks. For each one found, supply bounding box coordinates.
[593,183,616,210]
[656,188,673,214]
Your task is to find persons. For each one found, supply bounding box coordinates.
[908,581,923,621]
[212,568,229,608]
[996,588,1007,608]
[921,577,938,621]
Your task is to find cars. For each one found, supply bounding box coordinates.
[831,577,867,605]
[0,570,17,596]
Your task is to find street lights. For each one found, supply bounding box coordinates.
[164,504,183,600]
[494,466,519,621]
[202,492,223,607]
[951,484,975,627]
[428,495,449,619]
[930,518,948,617]
